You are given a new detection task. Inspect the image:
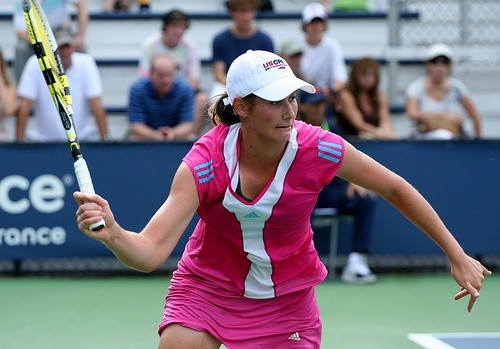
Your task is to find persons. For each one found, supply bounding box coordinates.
[16,24,109,142]
[278,40,304,79]
[295,84,379,283]
[0,45,16,116]
[124,54,195,142]
[12,0,86,116]
[405,44,482,140]
[333,59,395,140]
[283,3,349,103]
[137,10,210,141]
[209,0,275,106]
[73,49,491,349]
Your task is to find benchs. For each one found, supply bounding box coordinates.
[0,0,457,143]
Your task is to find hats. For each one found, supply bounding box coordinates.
[425,45,451,63]
[302,3,325,23]
[55,32,74,47]
[222,49,316,105]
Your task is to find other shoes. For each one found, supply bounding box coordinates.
[341,268,374,283]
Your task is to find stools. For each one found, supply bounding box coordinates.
[312,206,342,281]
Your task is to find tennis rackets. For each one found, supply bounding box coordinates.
[21,0,105,232]
